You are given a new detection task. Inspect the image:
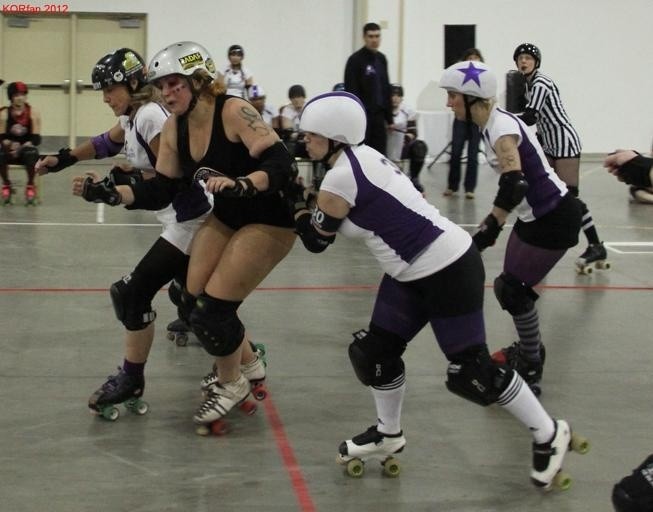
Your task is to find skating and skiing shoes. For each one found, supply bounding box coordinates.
[166,320,192,347]
[24,184,42,207]
[491,342,543,397]
[200,356,269,401]
[530,417,590,491]
[192,372,258,436]
[1,185,19,208]
[88,367,148,421]
[213,342,266,375]
[337,426,407,477]
[575,241,611,275]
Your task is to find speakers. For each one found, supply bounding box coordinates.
[443,23,477,72]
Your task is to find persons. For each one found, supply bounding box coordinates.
[221,44,253,101]
[71,39,306,438]
[0,82,44,207]
[512,42,611,277]
[438,60,582,399]
[390,82,429,203]
[286,90,590,495]
[601,145,653,206]
[30,46,213,420]
[442,48,486,200]
[342,22,392,158]
[248,84,281,133]
[281,85,328,193]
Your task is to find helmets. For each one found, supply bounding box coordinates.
[389,84,404,98]
[228,45,243,57]
[249,84,266,99]
[299,91,367,145]
[513,43,541,68]
[333,83,348,93]
[289,85,305,97]
[6,82,28,99]
[146,41,217,84]
[92,48,145,89]
[439,61,497,98]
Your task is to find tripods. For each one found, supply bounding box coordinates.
[426,139,487,171]
